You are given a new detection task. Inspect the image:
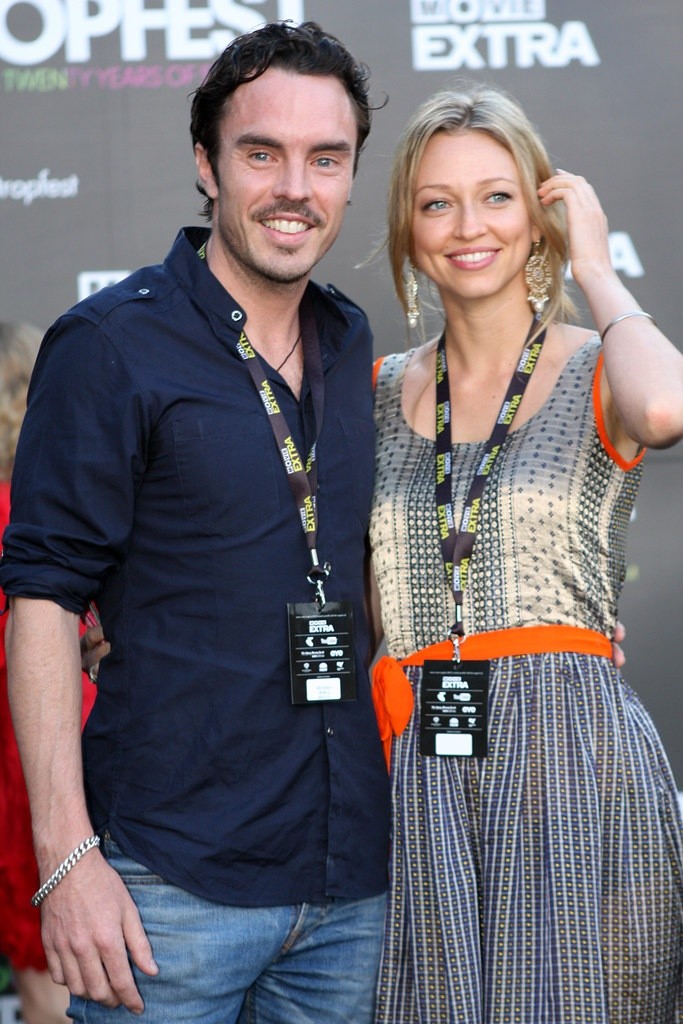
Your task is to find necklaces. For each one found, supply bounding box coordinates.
[270,328,305,377]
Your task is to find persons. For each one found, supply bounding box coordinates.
[0,22,626,1024]
[0,323,106,1023]
[81,89,683,1024]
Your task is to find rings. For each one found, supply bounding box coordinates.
[88,666,96,684]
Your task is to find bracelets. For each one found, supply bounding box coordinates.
[600,310,659,343]
[28,833,102,906]
